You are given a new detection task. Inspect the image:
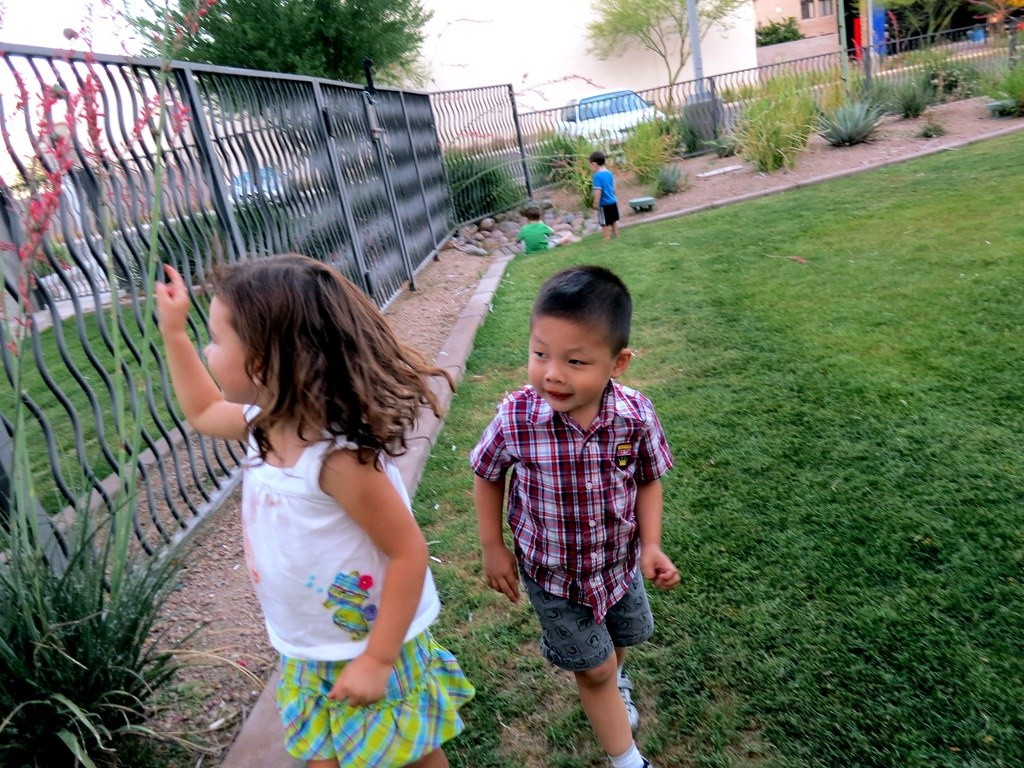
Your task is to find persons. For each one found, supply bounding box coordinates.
[468,264,681,768]
[589,151,620,240]
[153,251,478,768]
[517,209,570,255]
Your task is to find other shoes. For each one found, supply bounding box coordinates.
[617,665,640,736]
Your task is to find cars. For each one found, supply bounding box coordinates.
[228,164,298,208]
[556,90,675,157]
